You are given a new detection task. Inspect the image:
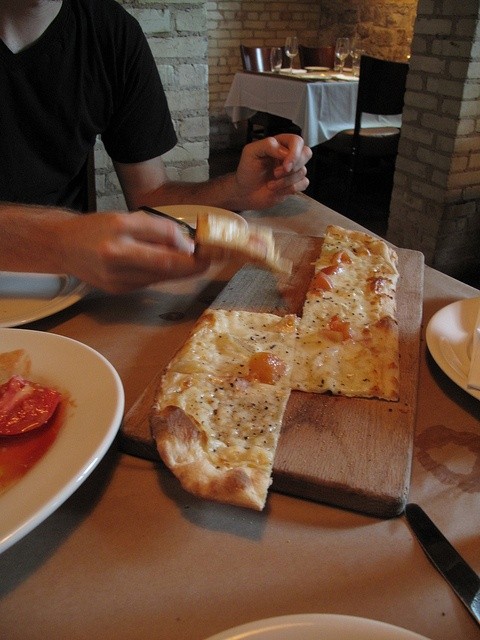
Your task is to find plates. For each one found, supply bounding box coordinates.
[422,293,480,405]
[333,74,360,82]
[0,325,127,551]
[0,269,91,326]
[204,612,432,638]
[143,203,250,241]
[306,65,333,72]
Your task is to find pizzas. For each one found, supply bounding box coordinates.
[293,222,400,402]
[193,214,291,276]
[150,298,299,511]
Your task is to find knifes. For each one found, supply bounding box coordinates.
[401,502,480,627]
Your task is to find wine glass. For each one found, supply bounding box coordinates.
[334,36,349,75]
[349,38,364,74]
[284,34,300,72]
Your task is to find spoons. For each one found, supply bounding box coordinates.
[139,205,198,238]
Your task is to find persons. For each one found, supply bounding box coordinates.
[0,0,314,294]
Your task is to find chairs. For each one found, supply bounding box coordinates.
[299,44,335,70]
[240,44,291,147]
[317,53,410,214]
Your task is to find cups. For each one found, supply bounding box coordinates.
[270,46,282,71]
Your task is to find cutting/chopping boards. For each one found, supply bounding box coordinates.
[120,229,427,522]
